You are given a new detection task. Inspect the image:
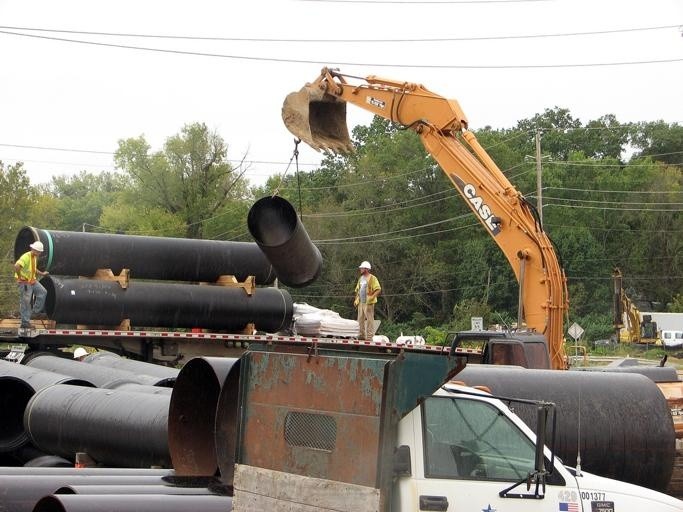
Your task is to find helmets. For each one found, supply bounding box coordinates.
[359,261,371,269]
[30,241,43,252]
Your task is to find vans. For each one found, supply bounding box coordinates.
[659,329,682,348]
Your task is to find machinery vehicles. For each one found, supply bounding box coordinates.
[278,67,683,440]
[606,265,663,348]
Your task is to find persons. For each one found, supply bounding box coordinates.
[72,345,88,362]
[12,240,49,330]
[353,260,381,342]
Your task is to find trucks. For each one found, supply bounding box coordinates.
[0,335,683,512]
